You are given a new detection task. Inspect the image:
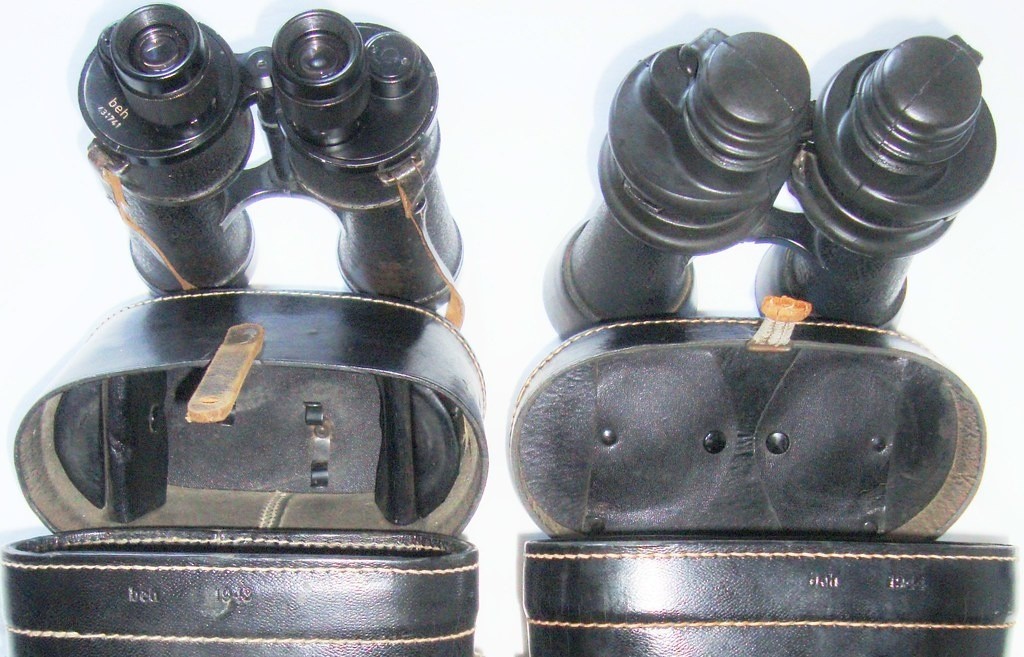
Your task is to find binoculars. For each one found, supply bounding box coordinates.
[543,25,997,348]
[77,4,464,310]
